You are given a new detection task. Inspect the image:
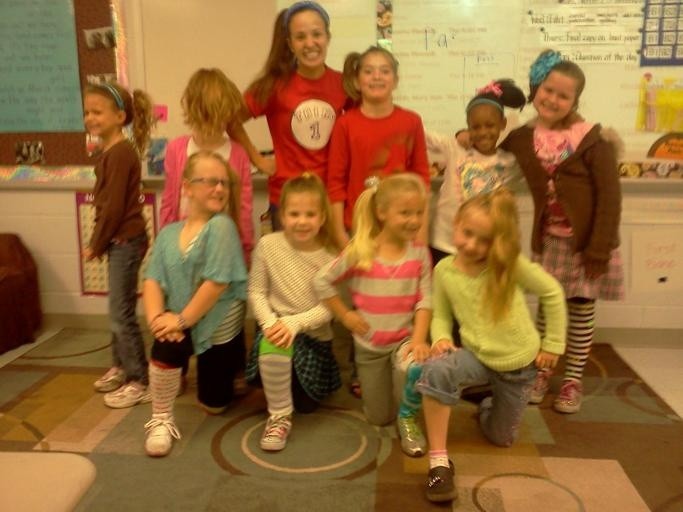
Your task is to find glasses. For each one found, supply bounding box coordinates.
[188,176,231,188]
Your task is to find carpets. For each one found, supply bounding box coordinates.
[1,326,682,512]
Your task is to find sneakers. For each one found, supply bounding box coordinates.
[460,383,494,405]
[261,413,293,451]
[553,377,585,414]
[397,414,427,457]
[103,382,154,408]
[144,411,181,458]
[93,367,128,392]
[520,368,554,404]
[425,458,458,502]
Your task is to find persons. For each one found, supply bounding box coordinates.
[82,82,155,409]
[456,60,622,413]
[325,46,431,400]
[245,172,340,452]
[159,67,254,273]
[142,147,249,457]
[312,172,435,456]
[415,183,569,503]
[423,78,536,347]
[233,1,364,234]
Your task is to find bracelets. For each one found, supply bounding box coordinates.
[177,313,189,330]
